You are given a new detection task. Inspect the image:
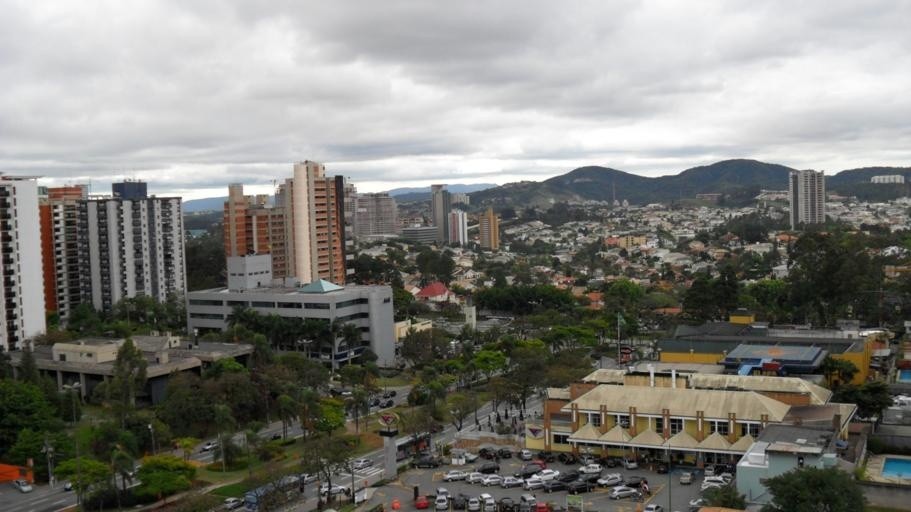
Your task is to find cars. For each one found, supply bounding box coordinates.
[223,458,375,512]
[63,480,80,491]
[201,440,218,452]
[128,464,142,478]
[13,479,32,493]
[368,390,397,408]
